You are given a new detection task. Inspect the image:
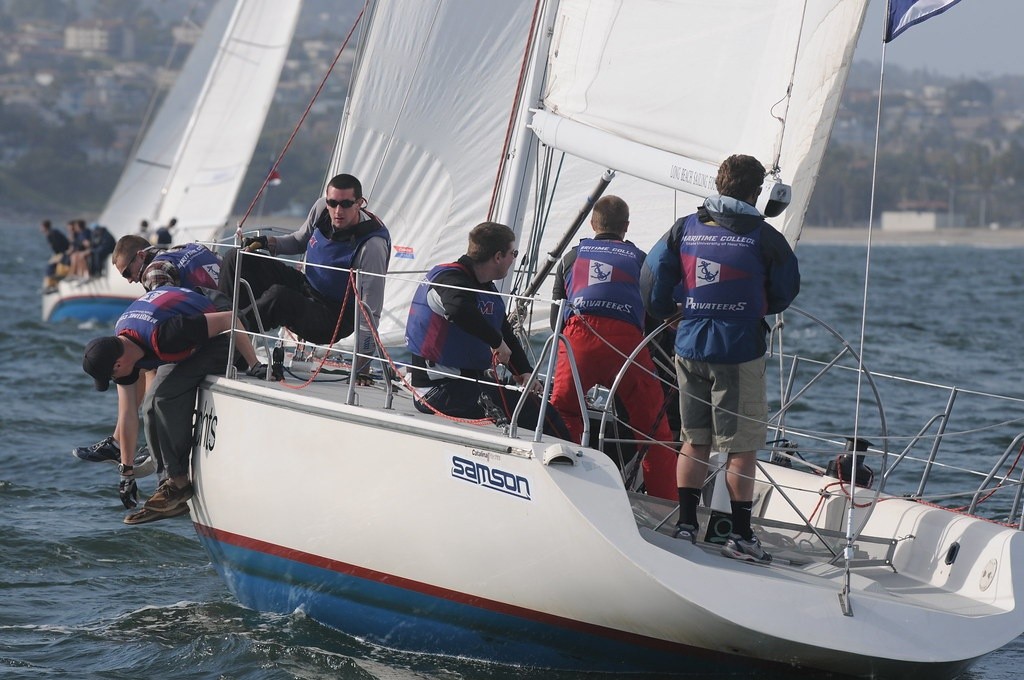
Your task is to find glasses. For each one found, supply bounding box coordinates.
[122,254,137,278]
[325,197,360,208]
[493,249,518,259]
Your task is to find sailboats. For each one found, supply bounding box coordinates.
[185,0,1023,680]
[31,0,305,322]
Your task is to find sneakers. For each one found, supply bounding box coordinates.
[124,502,190,524]
[117,446,155,478]
[143,480,194,511]
[673,520,699,544]
[73,436,121,465]
[721,533,772,564]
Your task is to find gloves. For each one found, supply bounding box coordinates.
[250,361,274,379]
[120,474,140,510]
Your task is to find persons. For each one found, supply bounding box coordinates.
[405,222,573,443]
[218,173,391,384]
[73,235,224,478]
[550,195,680,503]
[82,287,284,524]
[640,154,801,566]
[39,219,116,294]
[136,219,177,250]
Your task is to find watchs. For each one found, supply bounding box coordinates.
[118,463,133,473]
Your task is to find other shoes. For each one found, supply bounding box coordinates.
[63,272,80,282]
[42,284,59,295]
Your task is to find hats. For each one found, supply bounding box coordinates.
[83,336,123,391]
